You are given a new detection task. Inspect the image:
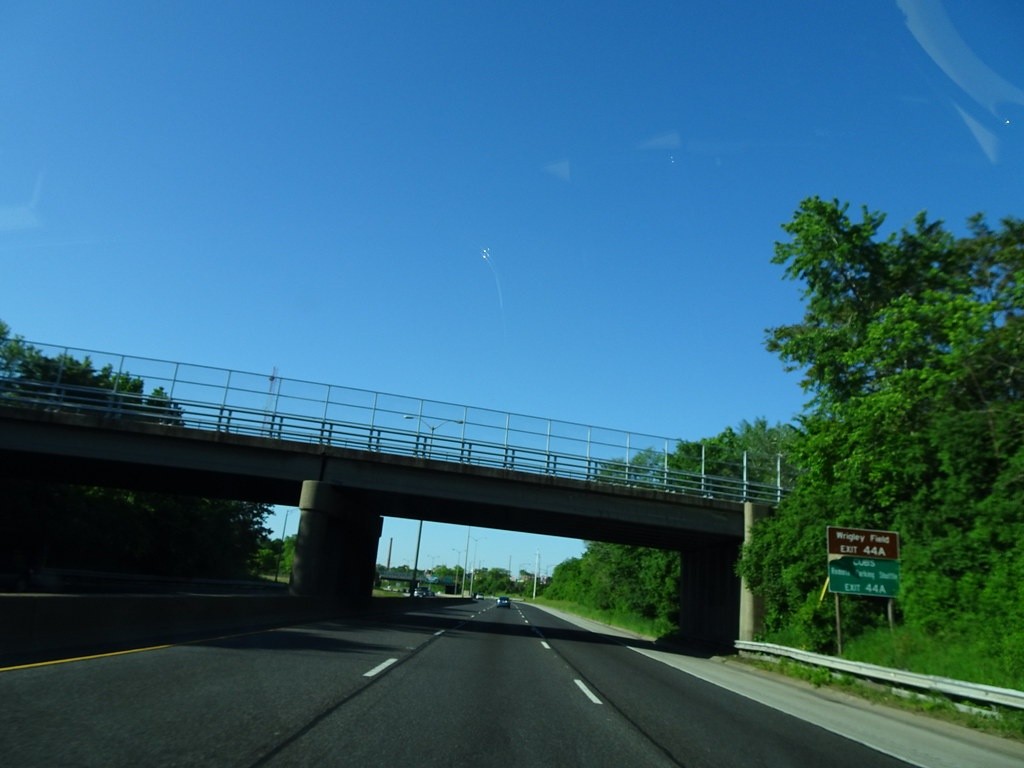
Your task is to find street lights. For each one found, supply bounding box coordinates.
[273,508,298,584]
[403,415,464,459]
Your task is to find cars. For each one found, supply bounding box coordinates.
[414,586,435,598]
[496,596,512,609]
[476,593,485,600]
